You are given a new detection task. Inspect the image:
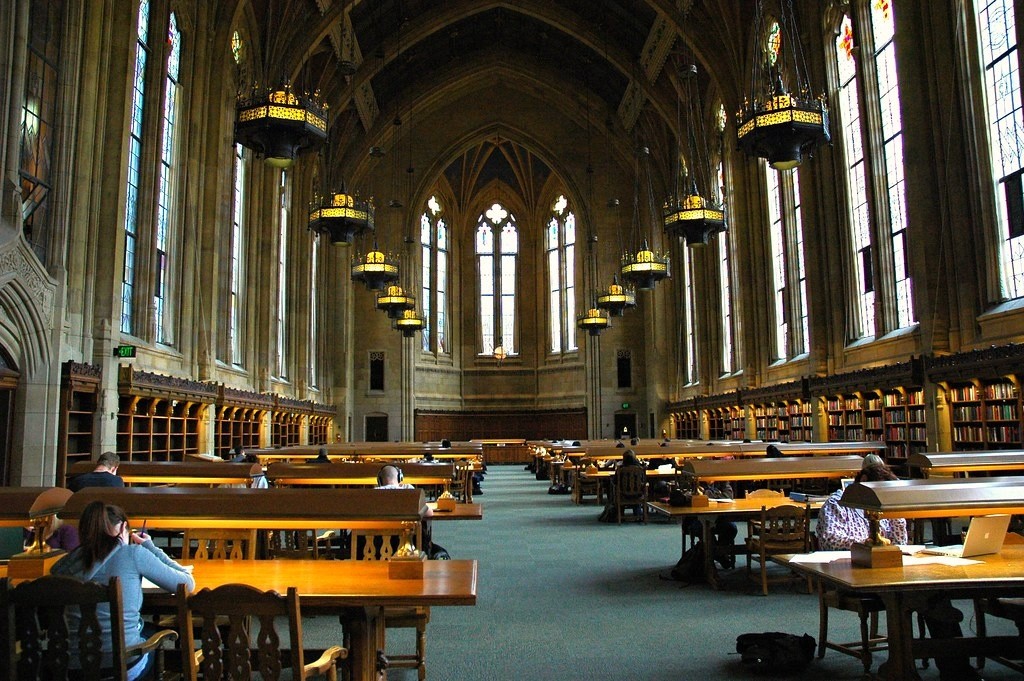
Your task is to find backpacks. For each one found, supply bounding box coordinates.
[736,632,817,676]
[670,541,707,585]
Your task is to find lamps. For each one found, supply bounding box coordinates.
[576,0,831,336]
[232,1,427,337]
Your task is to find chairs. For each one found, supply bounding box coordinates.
[1,575,184,681]
[339,522,431,681]
[152,528,257,673]
[264,529,336,617]
[448,461,469,503]
[810,530,930,676]
[744,503,813,595]
[176,583,348,681]
[610,466,649,524]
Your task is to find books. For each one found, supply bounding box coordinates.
[724,381,1018,457]
[789,492,828,503]
[894,545,925,554]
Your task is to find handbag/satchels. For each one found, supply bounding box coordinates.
[598,501,619,523]
[669,487,686,507]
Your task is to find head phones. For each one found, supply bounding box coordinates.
[377,465,403,487]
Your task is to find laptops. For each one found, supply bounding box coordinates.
[841,479,854,491]
[920,513,1012,556]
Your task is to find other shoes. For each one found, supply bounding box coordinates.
[716,542,732,569]
[939,666,982,681]
[621,514,625,523]
[720,539,735,567]
[635,513,644,522]
[915,598,964,623]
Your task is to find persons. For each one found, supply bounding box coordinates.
[420,439,455,463]
[217,446,268,488]
[731,439,752,459]
[50,502,196,681]
[631,437,671,447]
[604,443,625,467]
[816,454,982,681]
[67,451,125,493]
[761,446,785,489]
[677,475,738,569]
[701,443,721,460]
[374,464,425,550]
[306,448,331,463]
[647,443,684,498]
[612,450,644,519]
[12,514,81,640]
[535,439,581,488]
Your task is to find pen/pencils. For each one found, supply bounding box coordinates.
[139,518,147,540]
[902,551,912,556]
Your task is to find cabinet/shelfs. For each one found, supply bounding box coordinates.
[483,445,535,465]
[56,359,339,488]
[670,367,1024,457]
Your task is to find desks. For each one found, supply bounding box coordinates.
[0,442,484,681]
[526,440,1024,681]
